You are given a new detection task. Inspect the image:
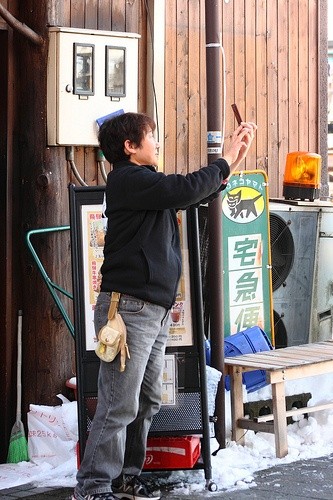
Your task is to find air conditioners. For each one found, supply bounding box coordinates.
[198,199,333,348]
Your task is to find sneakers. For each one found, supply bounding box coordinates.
[72,477,165,500]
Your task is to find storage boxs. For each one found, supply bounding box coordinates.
[75,434,200,470]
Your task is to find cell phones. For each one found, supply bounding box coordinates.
[231,104,242,126]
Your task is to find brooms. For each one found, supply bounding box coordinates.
[5,311,30,464]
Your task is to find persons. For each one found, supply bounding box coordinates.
[69,113,257,500]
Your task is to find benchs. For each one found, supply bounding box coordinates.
[224,340,333,458]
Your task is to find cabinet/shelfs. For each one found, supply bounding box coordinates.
[68,183,218,491]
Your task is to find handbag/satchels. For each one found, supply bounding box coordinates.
[91,308,136,373]
